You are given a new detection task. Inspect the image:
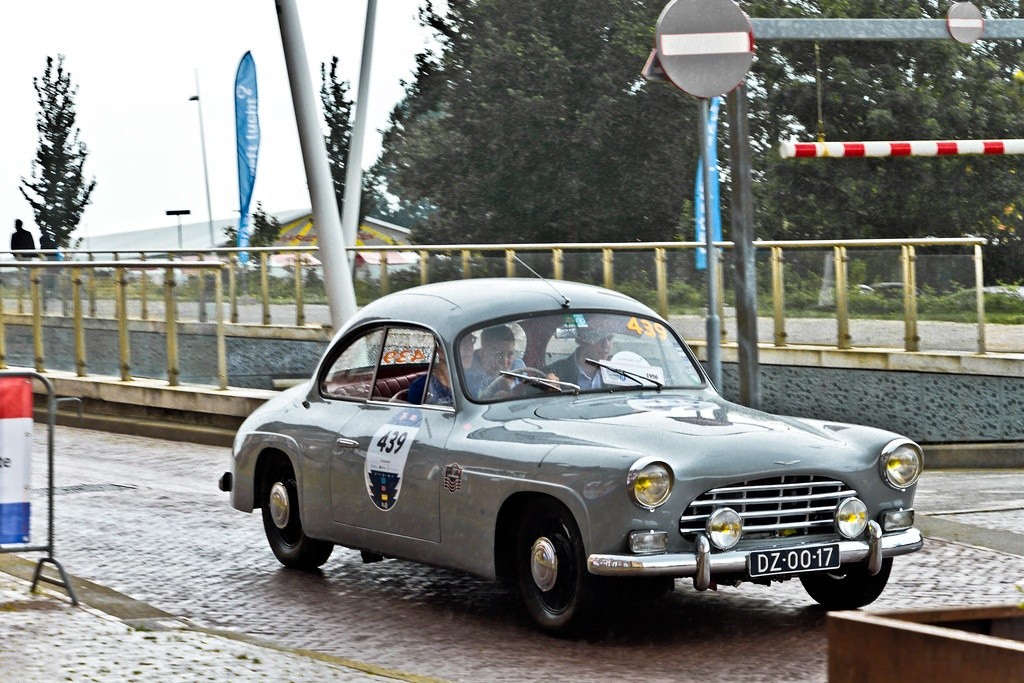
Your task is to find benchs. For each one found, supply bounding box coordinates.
[334,371,429,401]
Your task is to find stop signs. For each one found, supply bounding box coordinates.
[655,0,755,98]
[946,2,985,43]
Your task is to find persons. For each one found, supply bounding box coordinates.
[536,319,615,390]
[10,219,39,258]
[466,324,527,400]
[396,331,561,406]
[40,227,62,296]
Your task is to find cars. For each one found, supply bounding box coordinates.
[945,287,1024,305]
[220,273,923,641]
[848,282,926,305]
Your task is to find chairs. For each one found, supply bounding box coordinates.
[390,389,408,403]
